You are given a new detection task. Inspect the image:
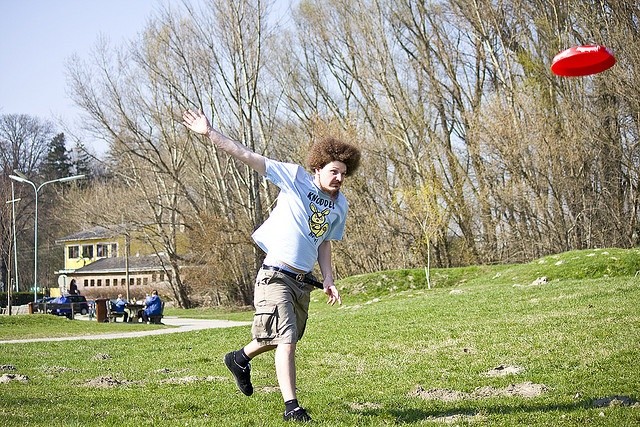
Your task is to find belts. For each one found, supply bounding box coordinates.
[263,264,323,289]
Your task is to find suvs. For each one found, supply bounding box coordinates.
[46,295,89,316]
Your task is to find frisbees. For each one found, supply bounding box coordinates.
[551,45,617,76]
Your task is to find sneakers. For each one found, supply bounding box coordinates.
[225,351,253,396]
[283,407,313,422]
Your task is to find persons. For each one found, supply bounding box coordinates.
[139,290,161,323]
[69,278,78,296]
[114,293,129,321]
[182,107,360,423]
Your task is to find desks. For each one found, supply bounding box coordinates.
[126,302,144,324]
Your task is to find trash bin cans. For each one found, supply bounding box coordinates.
[94,298,109,323]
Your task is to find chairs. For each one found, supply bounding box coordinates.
[148,300,166,324]
[105,299,124,324]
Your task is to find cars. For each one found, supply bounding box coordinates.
[34,297,56,312]
